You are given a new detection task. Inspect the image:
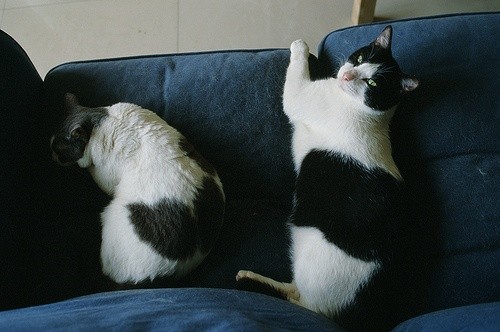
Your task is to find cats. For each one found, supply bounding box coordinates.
[48,91,226,290]
[234,26,417,321]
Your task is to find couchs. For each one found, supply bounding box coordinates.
[0,11,500,332]
